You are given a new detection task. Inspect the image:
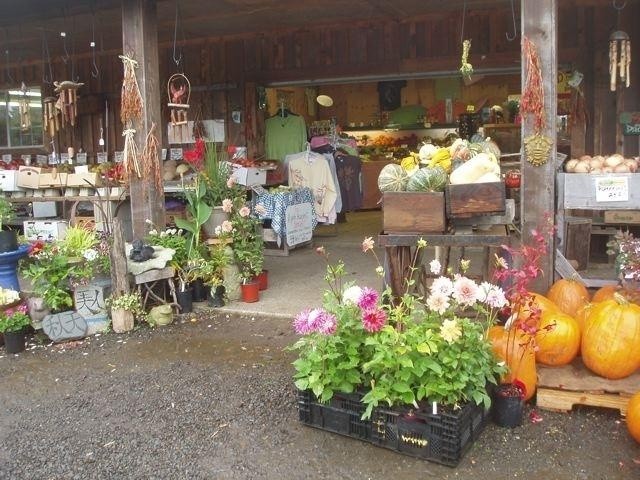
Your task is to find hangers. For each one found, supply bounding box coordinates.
[277,98,348,164]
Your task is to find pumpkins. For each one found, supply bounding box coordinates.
[487,277,640,445]
[377,134,501,192]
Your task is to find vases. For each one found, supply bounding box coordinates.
[297,372,503,470]
[490,381,526,428]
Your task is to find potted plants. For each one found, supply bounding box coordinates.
[1,124,270,353]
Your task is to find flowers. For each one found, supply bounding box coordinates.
[488,207,561,395]
[278,227,515,423]
[606,222,640,305]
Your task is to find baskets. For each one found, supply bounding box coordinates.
[298,385,492,467]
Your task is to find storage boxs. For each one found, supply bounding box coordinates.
[555,173,640,211]
[445,180,508,221]
[383,190,448,234]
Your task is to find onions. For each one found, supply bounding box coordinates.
[566,154,640,173]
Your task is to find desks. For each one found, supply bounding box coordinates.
[378,224,512,334]
